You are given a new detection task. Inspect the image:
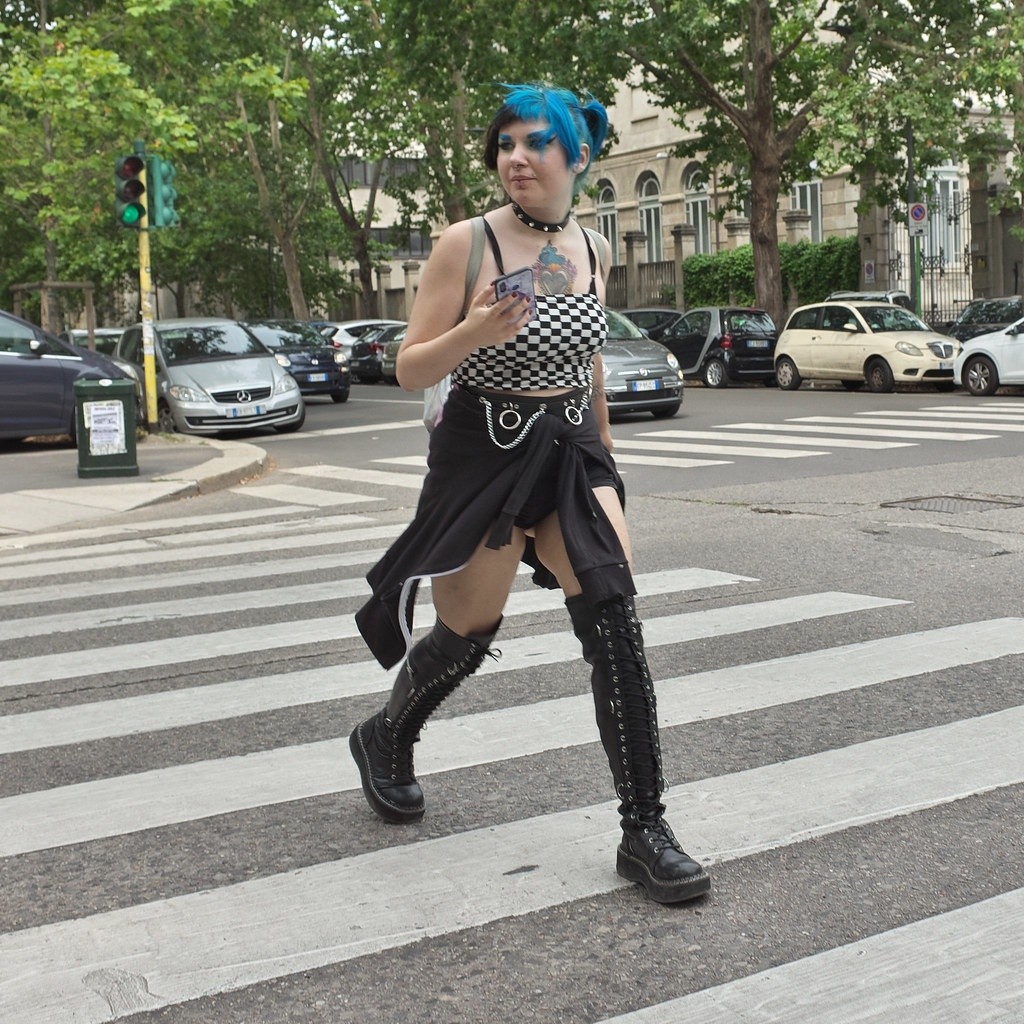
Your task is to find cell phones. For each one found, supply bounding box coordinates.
[494,266,538,324]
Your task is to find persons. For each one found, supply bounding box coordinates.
[349,87,711,904]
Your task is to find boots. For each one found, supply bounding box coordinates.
[563,590,710,901]
[349,611,504,822]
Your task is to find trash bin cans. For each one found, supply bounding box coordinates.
[71,375,142,480]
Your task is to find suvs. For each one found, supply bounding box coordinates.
[312,319,408,384]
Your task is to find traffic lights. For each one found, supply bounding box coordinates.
[113,156,145,228]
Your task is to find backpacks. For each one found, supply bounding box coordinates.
[422,217,608,435]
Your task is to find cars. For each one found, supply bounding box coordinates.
[952,316,1024,396]
[946,295,1024,344]
[237,320,354,405]
[824,290,910,309]
[600,305,684,420]
[773,300,963,390]
[0,308,146,447]
[50,327,125,357]
[656,308,777,389]
[110,317,307,438]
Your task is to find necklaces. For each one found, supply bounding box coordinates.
[513,202,571,233]
